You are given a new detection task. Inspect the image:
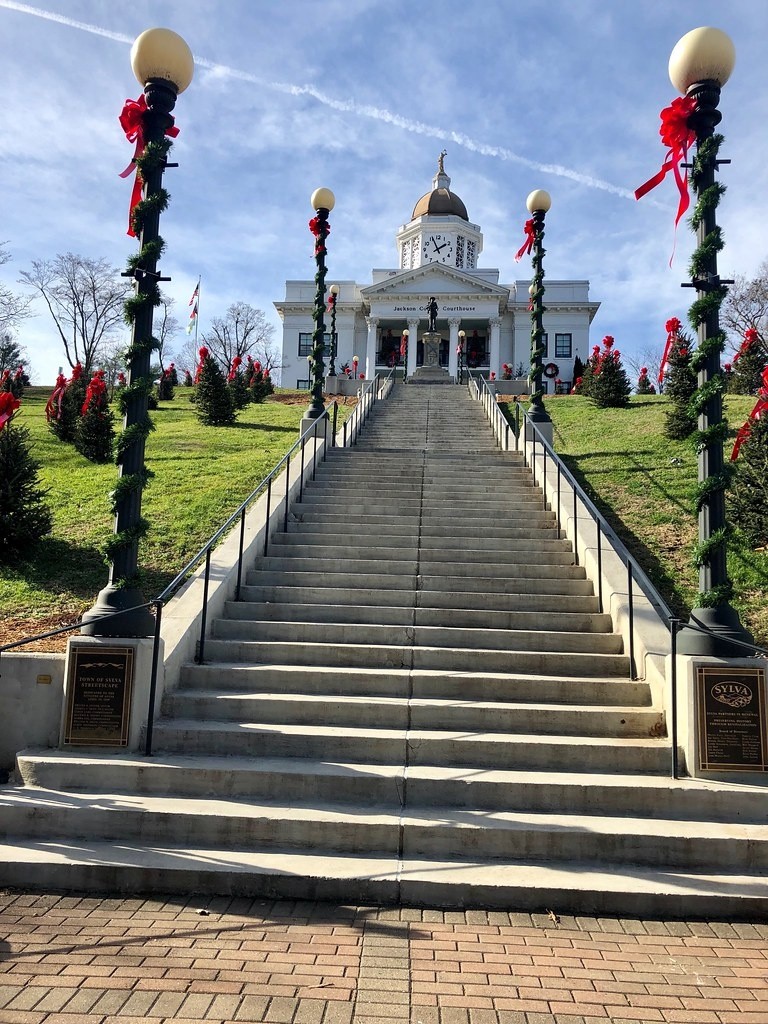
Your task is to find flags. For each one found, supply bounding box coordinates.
[187,318,195,336]
[189,283,199,306]
[190,302,198,319]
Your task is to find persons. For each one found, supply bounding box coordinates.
[427,296,438,331]
[437,152,447,172]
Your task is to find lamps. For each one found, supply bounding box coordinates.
[353,356,359,361]
[508,363,513,369]
[307,355,313,362]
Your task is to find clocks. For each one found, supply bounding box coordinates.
[423,234,453,263]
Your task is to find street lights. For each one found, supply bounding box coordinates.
[306,355,312,390]
[302,188,336,420]
[523,188,552,421]
[457,330,465,385]
[353,356,359,379]
[326,285,340,375]
[403,329,410,384]
[668,29,757,656]
[78,27,194,637]
